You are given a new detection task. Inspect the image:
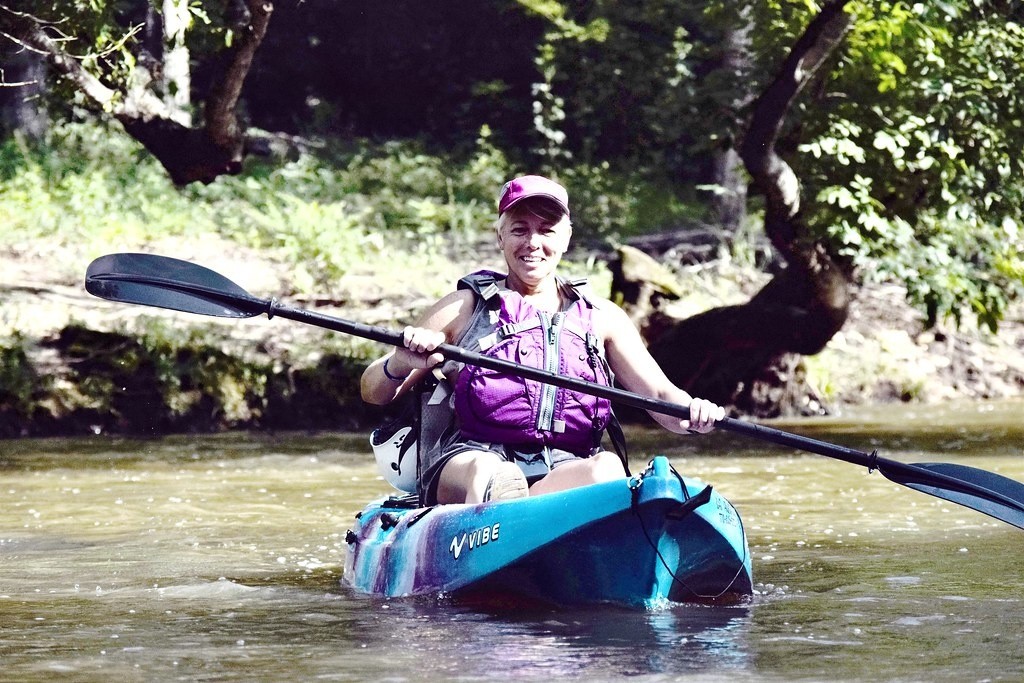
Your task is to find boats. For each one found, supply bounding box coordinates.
[343,453,753,608]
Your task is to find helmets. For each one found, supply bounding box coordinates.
[369,427,417,493]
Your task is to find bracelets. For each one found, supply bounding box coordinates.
[383,358,405,382]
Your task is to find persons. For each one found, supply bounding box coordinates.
[361,175,725,504]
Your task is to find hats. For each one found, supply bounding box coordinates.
[499,175,570,216]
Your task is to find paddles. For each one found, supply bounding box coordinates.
[84,252,1024,530]
